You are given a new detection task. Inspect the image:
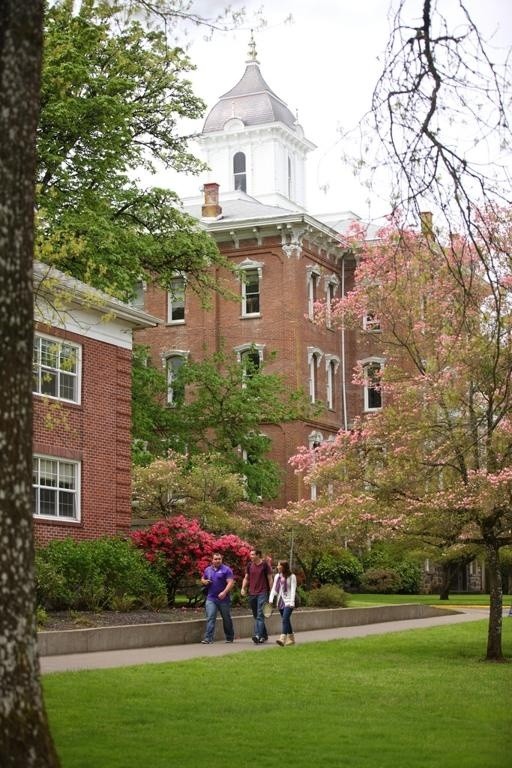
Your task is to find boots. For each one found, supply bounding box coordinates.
[276,633,285,647]
[285,632,295,646]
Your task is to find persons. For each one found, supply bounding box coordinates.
[269,559,300,646]
[199,553,235,644]
[240,548,274,644]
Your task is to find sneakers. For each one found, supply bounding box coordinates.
[252,635,267,644]
[201,639,209,644]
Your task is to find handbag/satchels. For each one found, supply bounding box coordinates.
[200,586,208,595]
